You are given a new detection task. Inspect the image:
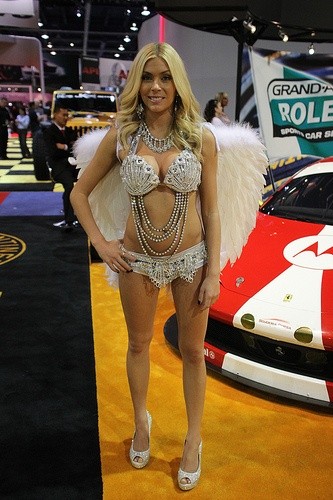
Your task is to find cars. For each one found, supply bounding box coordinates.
[162,154,333,413]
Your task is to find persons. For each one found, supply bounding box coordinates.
[41,106,79,224]
[28,102,39,137]
[15,107,31,158]
[69,40,222,491]
[204,92,231,129]
[0,96,14,160]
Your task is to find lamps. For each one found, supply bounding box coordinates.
[272,25,289,42]
[307,39,315,55]
[242,12,256,33]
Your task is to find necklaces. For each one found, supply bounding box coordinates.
[141,121,175,153]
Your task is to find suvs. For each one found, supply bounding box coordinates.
[31,88,118,181]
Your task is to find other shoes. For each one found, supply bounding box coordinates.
[130,409,152,469]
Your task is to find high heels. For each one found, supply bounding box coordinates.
[176,438,203,491]
